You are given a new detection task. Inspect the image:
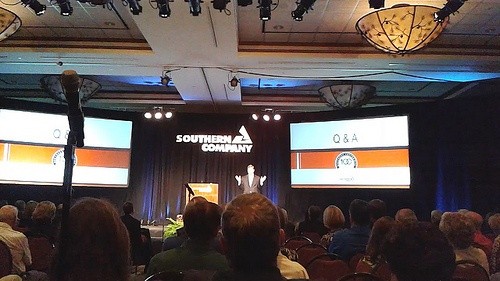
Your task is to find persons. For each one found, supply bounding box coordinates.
[235,165,267,194]
[0,194,500,281]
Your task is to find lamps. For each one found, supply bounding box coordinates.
[317,83,377,113]
[39,73,103,105]
[161,69,171,89]
[148,0,174,19]
[20,0,46,17]
[433,0,466,24]
[256,0,280,22]
[355,3,450,58]
[51,0,74,17]
[121,0,142,16]
[228,72,241,90]
[184,0,204,17]
[291,0,315,22]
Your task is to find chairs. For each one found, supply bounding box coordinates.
[282,235,383,281]
[28,232,54,272]
[453,260,490,281]
[0,240,13,279]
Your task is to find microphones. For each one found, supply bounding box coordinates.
[185,183,194,196]
[59,69,84,147]
